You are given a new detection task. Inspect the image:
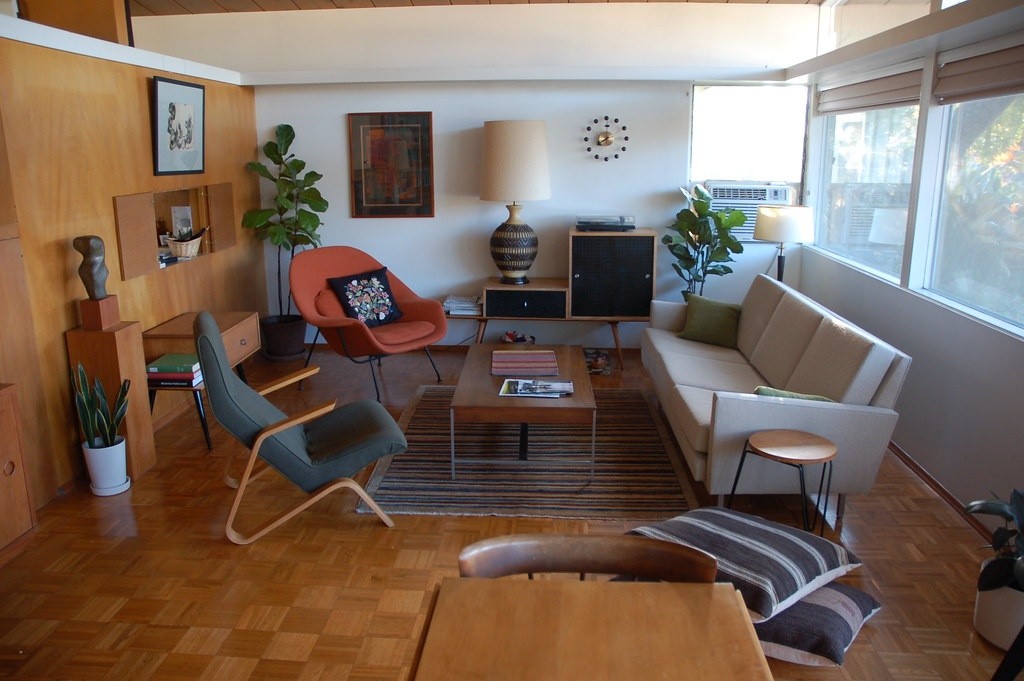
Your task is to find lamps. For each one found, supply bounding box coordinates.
[480,120,551,284]
[752,205,815,282]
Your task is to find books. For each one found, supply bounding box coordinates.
[444,294,484,315]
[491,349,559,377]
[575,215,635,232]
[146,353,204,388]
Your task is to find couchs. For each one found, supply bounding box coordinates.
[641,273,915,512]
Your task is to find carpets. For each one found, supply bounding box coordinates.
[354,386,700,520]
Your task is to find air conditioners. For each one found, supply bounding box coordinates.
[704,180,790,242]
[841,184,912,248]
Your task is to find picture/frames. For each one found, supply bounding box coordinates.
[347,111,435,218]
[152,75,205,175]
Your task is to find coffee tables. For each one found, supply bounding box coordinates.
[448,344,598,480]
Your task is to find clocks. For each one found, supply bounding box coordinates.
[583,116,629,161]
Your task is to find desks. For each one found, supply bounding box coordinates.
[141,310,262,450]
[413,577,774,681]
[727,429,838,537]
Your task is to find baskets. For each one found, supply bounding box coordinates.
[165,236,202,258]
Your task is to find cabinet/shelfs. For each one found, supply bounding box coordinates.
[0,382,38,566]
[443,225,657,371]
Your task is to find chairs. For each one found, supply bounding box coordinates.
[289,247,448,401]
[193,311,409,546]
[458,533,719,585]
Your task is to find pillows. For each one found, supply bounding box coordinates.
[676,293,741,349]
[754,385,837,403]
[314,290,347,318]
[607,574,882,668]
[324,266,405,328]
[621,507,863,622]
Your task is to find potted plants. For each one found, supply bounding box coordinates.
[69,361,132,496]
[962,487,1024,681]
[240,123,330,358]
